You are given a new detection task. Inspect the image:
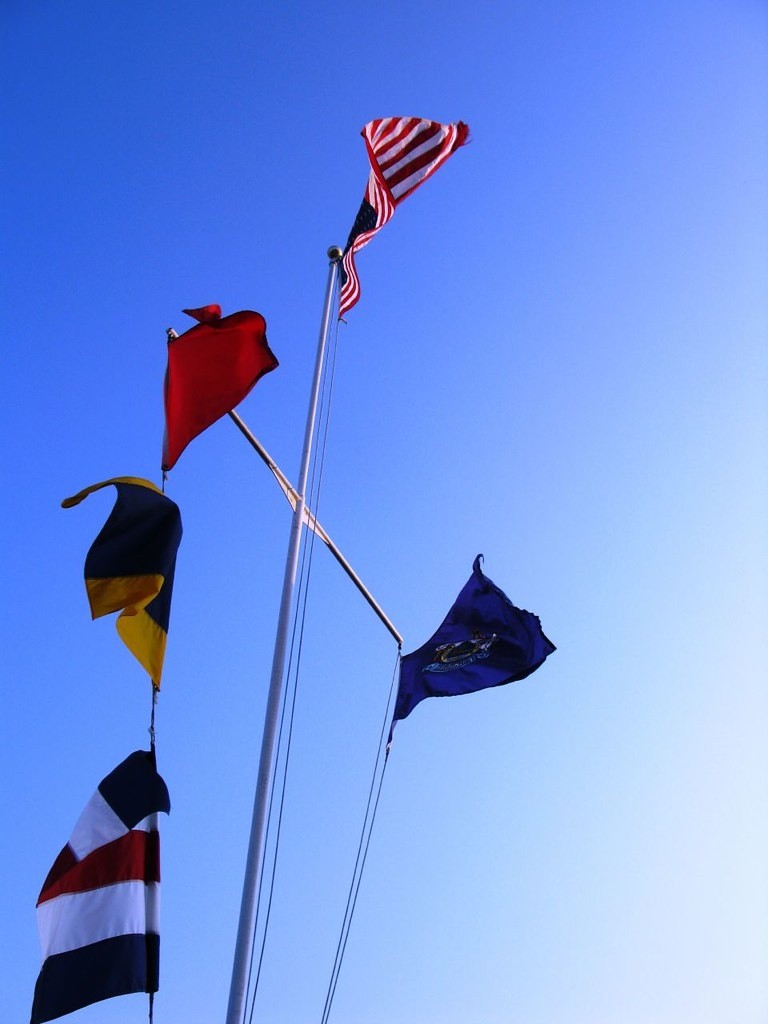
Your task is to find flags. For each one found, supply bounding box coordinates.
[31,743,171,1024]
[337,115,470,318]
[62,476,183,694]
[162,304,279,472]
[385,554,557,763]
